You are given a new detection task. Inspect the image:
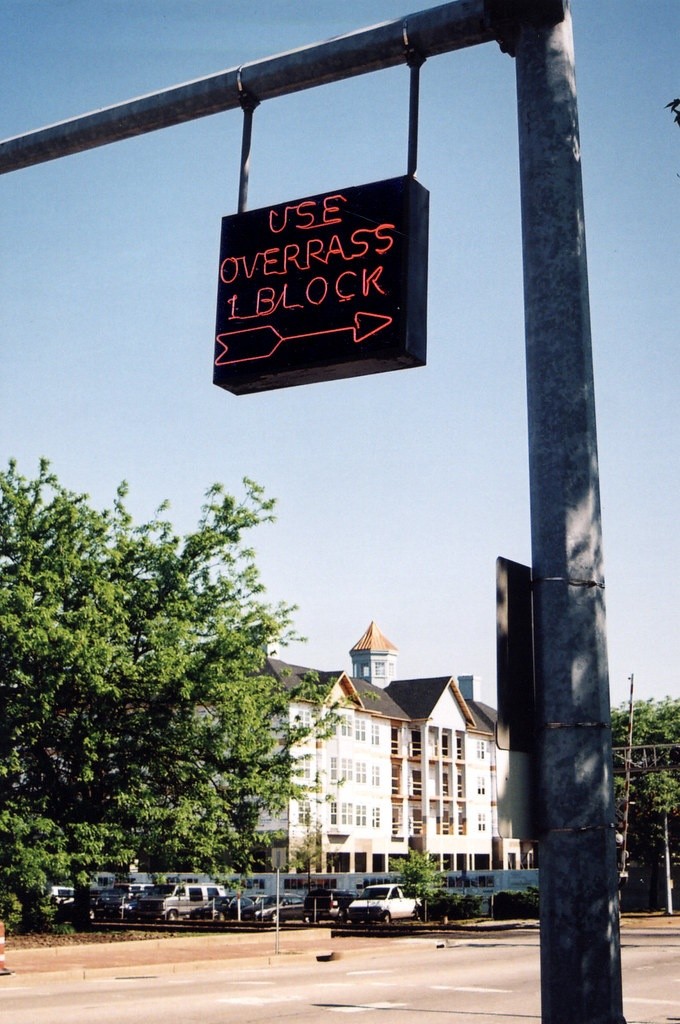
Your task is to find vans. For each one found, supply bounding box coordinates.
[136,882,225,921]
[304,888,360,925]
[347,884,424,923]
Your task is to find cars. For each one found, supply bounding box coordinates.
[191,895,257,920]
[50,883,157,922]
[242,892,307,924]
